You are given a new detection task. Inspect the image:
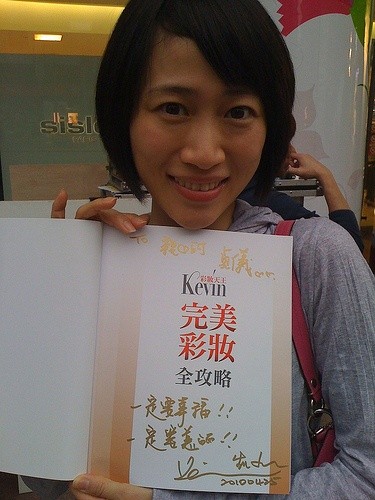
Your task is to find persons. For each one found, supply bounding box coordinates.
[50,0,375,500]
[269,143,365,254]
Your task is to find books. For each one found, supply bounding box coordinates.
[1,217,294,498]
